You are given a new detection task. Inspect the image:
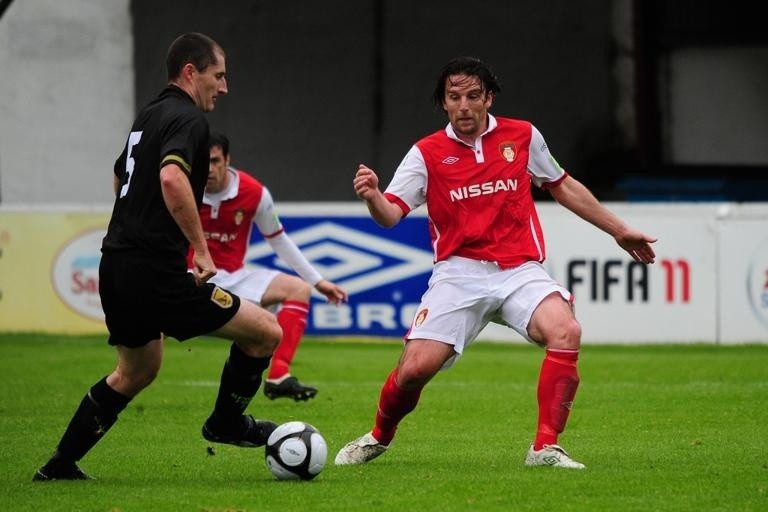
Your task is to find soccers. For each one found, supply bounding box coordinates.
[265,420,329,481]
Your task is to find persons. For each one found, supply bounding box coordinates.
[29,31,282,480]
[333,55,658,470]
[186,130,348,402]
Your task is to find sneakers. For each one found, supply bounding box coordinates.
[31,465,97,481]
[201,414,278,449]
[523,444,585,470]
[334,430,390,466]
[263,376,318,403]
